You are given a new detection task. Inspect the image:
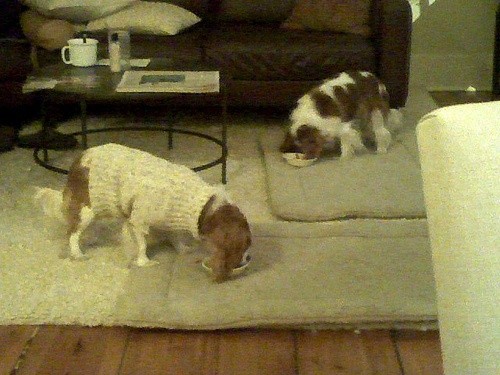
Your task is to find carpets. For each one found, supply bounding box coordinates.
[258,128,428,221]
[0,88,445,334]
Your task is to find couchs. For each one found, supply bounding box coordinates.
[0,0,413,152]
[418,101,500,375]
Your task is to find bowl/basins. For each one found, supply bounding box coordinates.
[283,153,317,168]
[202,257,253,275]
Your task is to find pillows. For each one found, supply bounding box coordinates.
[22,0,200,50]
[215,3,369,37]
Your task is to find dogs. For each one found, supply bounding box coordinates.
[34,144,252,282]
[278,71,403,164]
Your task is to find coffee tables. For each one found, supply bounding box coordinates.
[24,61,228,183]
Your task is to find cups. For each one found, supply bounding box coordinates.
[61,38,99,68]
[108,30,131,73]
